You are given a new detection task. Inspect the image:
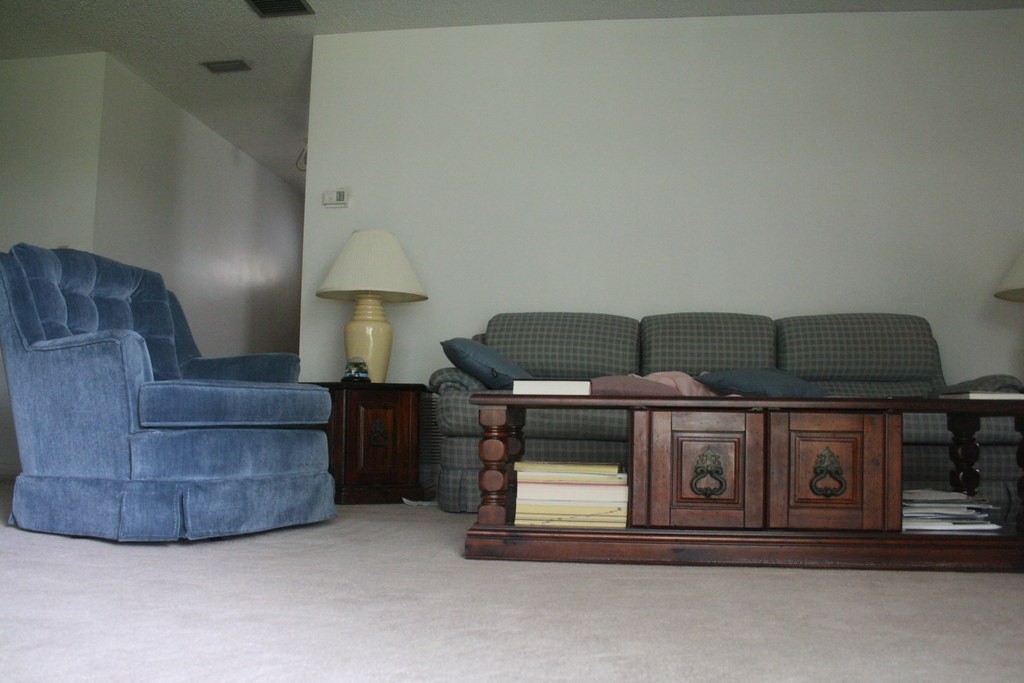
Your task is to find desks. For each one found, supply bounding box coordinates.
[463,392,1024,573]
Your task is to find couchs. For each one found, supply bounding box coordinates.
[426,313,1024,516]
[0,244,338,544]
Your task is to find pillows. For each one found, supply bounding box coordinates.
[440,338,533,390]
[693,367,825,401]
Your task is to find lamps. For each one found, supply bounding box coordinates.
[315,229,429,383]
[994,248,1024,302]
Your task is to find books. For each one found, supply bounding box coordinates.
[512,459,628,530]
[900,489,1002,529]
[938,390,1024,401]
[510,379,591,395]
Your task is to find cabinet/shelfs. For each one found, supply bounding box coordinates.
[300,382,427,506]
